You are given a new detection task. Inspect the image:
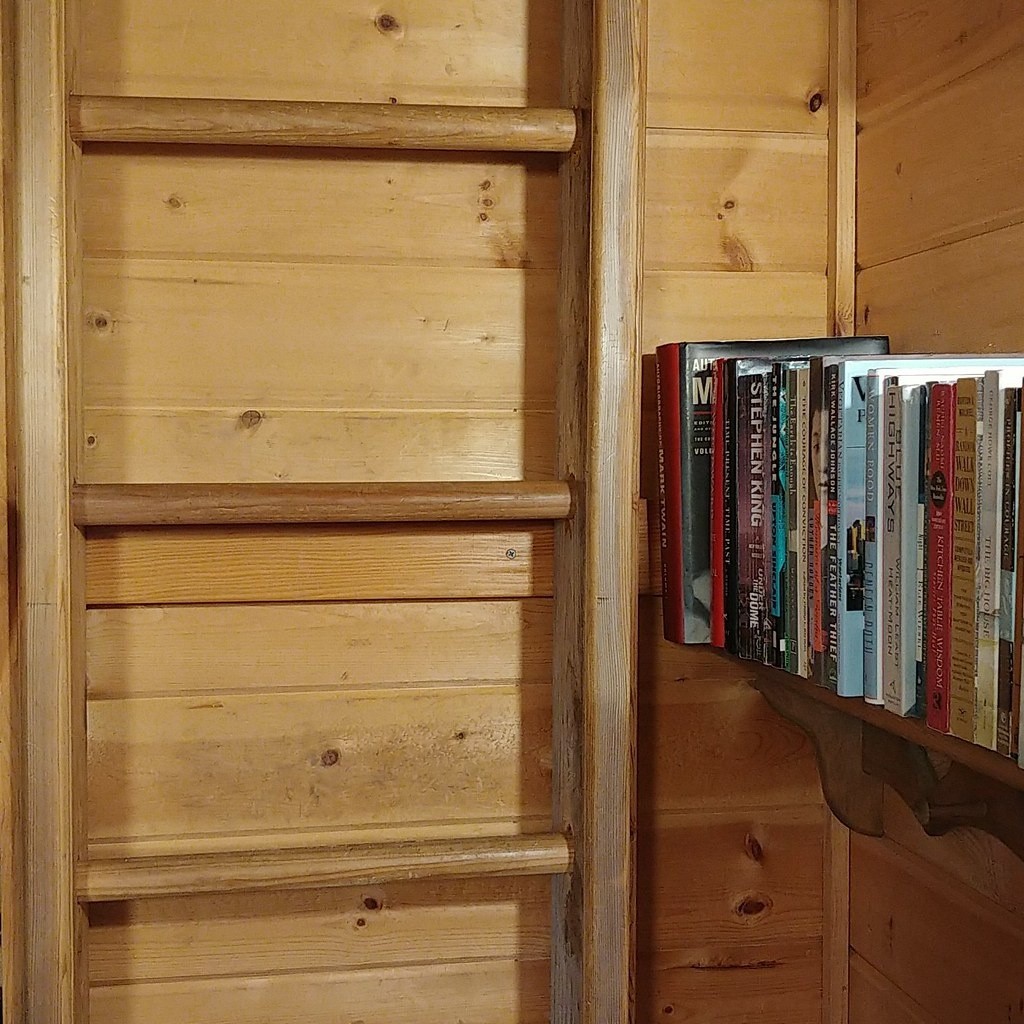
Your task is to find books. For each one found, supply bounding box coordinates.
[641,333,1024,764]
